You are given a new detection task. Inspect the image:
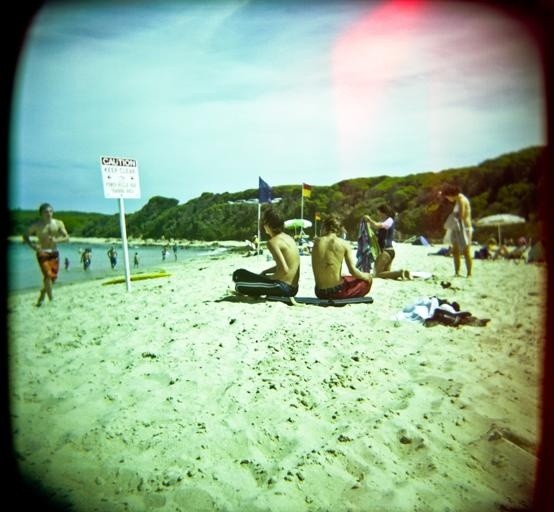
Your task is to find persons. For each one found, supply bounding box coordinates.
[362,203,414,281]
[340,225,347,239]
[231,208,301,302]
[78,248,91,271]
[492,237,527,262]
[425,245,452,257]
[107,247,118,269]
[23,203,68,309]
[439,183,474,278]
[473,238,496,260]
[251,234,258,246]
[307,217,373,299]
[296,237,316,255]
[160,242,178,262]
[133,252,139,268]
[63,257,69,270]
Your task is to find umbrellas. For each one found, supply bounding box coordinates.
[282,218,312,237]
[474,213,527,244]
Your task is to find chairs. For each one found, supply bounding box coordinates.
[505,237,532,265]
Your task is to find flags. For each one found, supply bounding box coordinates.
[257,177,272,206]
[303,183,312,199]
